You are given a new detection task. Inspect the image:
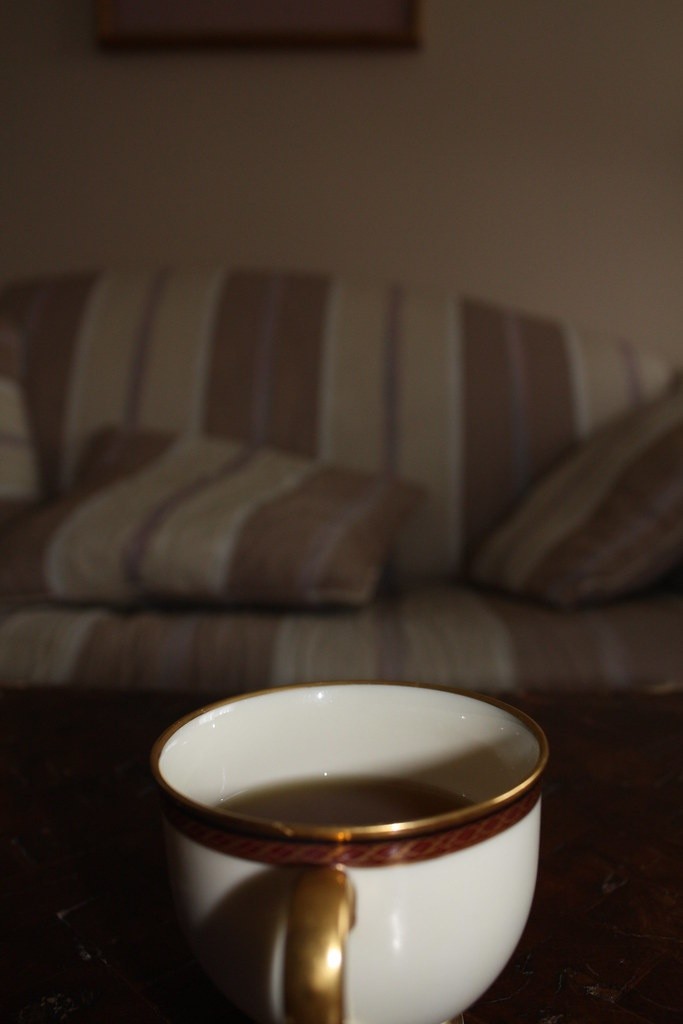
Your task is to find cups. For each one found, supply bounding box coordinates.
[150,680,548,1024]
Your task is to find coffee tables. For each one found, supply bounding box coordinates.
[0,691,683,1024]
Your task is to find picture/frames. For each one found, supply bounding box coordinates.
[95,0,424,46]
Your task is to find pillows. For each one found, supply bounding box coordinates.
[466,364,683,607]
[0,420,424,613]
[0,375,54,515]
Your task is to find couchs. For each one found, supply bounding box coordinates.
[0,261,683,686]
